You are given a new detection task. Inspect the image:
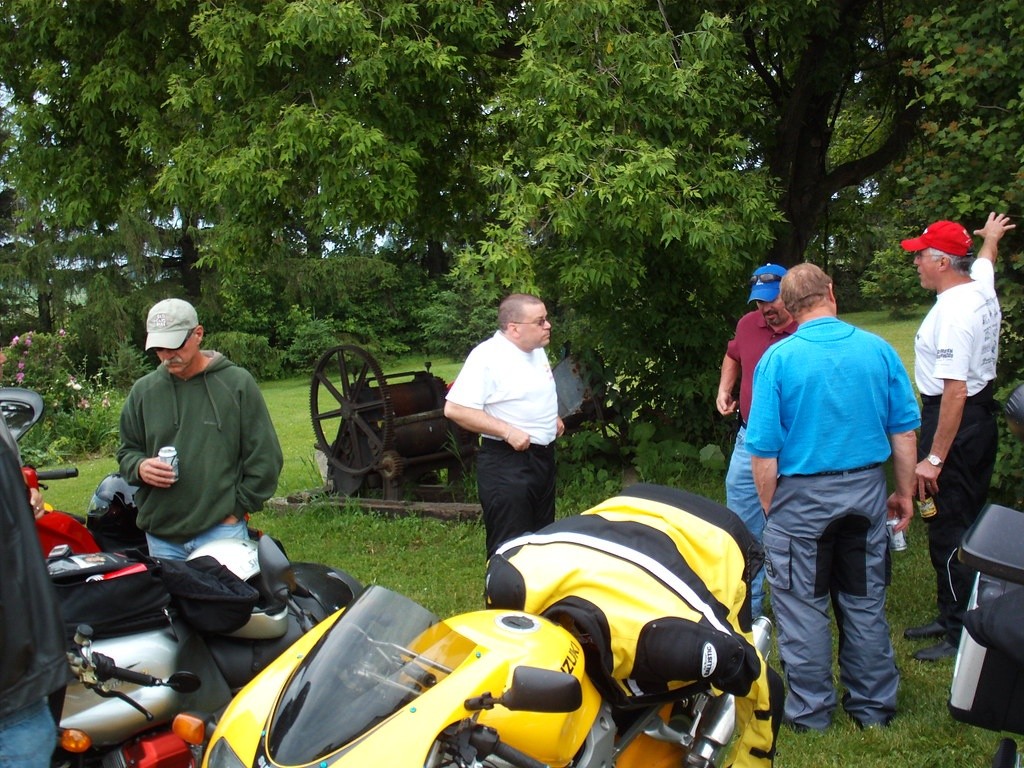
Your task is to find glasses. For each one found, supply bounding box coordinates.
[505,316,552,326]
[152,331,194,350]
[745,274,782,286]
[916,253,946,260]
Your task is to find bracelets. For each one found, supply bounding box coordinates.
[505,426,514,442]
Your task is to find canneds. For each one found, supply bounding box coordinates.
[886,518,907,551]
[158,446,180,482]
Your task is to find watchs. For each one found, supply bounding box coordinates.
[926,454,944,469]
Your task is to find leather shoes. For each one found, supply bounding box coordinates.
[903,622,949,641]
[913,640,957,662]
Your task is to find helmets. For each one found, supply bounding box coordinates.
[185,538,288,639]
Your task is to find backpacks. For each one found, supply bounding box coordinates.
[44,547,178,639]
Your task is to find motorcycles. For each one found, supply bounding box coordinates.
[0,386,363,768]
[943,381,1024,767]
[169,481,778,768]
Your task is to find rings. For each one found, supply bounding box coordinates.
[926,482,931,486]
[35,507,40,513]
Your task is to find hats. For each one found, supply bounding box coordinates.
[143,298,199,349]
[902,221,972,257]
[747,265,788,304]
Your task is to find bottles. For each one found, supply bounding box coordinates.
[915,484,940,523]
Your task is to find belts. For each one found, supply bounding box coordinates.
[741,421,748,430]
[921,385,988,405]
[790,462,881,479]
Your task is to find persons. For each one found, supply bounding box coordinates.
[0,412,69,768]
[715,263,799,622]
[116,298,284,583]
[444,294,565,560]
[901,212,1016,664]
[744,262,922,737]
